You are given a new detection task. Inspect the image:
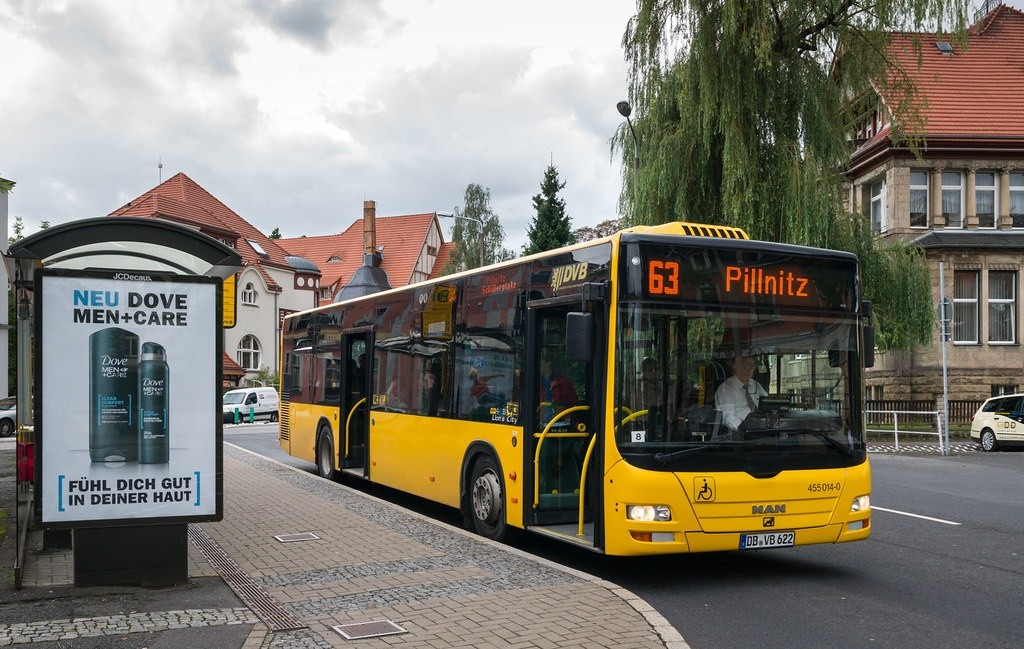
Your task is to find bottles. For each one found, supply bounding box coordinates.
[138,342,170,465]
[88,327,141,463]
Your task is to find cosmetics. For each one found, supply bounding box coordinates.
[88,327,170,465]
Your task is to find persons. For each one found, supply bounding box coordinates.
[468,368,506,408]
[639,358,659,389]
[537,354,578,423]
[714,353,769,432]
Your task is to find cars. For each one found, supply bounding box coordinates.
[970,393,1024,452]
[0,397,33,438]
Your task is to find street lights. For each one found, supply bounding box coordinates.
[437,211,484,269]
[616,101,639,203]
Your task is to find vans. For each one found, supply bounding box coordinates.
[222,386,281,422]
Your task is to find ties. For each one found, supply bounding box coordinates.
[742,384,757,412]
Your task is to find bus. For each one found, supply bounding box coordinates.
[277,220,874,557]
